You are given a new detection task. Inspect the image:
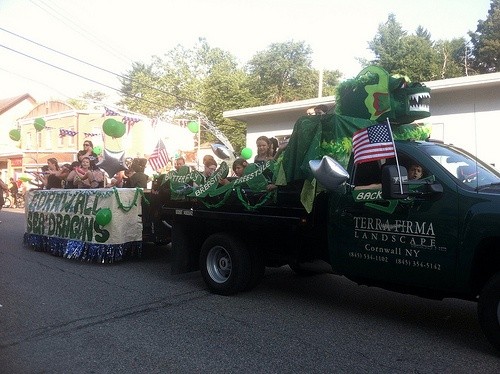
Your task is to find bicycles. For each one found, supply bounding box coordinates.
[2,192,25,209]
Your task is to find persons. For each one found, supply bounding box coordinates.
[176,155,229,187]
[0,172,18,221]
[408,164,422,180]
[306,106,327,115]
[233,136,279,176]
[33,140,148,189]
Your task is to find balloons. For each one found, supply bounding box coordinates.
[188,122,198,133]
[103,119,126,138]
[8,129,21,141]
[241,147,253,159]
[33,117,46,130]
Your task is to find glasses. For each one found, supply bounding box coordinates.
[83,144,90,146]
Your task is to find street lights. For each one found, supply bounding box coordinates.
[76,112,90,150]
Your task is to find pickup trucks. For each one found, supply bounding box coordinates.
[161,138,500,353]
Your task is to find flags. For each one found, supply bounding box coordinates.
[149,140,169,169]
[353,122,395,164]
